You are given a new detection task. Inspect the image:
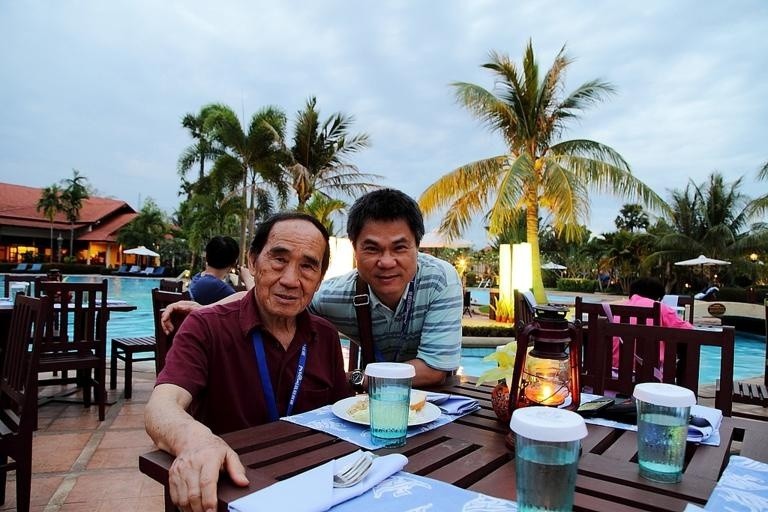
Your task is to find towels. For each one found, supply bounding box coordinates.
[426,393,480,416]
[244,452,409,512]
[686,405,723,441]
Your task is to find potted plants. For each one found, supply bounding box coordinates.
[475,340,534,423]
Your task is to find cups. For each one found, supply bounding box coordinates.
[509,407,588,511]
[364,362,417,444]
[10,281,30,302]
[630,382,696,486]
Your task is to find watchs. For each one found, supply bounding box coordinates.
[349,366,366,395]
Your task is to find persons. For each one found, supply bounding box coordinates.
[596,266,623,294]
[141,209,353,512]
[607,275,693,380]
[160,189,463,385]
[186,233,255,306]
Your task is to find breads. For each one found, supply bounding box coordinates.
[409,391,427,410]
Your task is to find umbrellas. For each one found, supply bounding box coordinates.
[675,253,731,290]
[123,246,160,265]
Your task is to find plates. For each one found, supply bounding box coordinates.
[333,397,442,427]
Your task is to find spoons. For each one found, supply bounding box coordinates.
[428,394,453,405]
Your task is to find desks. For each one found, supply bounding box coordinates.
[139,376,768,512]
[0,300,137,409]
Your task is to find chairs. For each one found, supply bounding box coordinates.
[514,289,735,417]
[28,278,108,432]
[110,279,182,401]
[2,276,72,385]
[151,288,193,381]
[0,289,50,511]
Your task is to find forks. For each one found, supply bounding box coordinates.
[334,452,380,489]
